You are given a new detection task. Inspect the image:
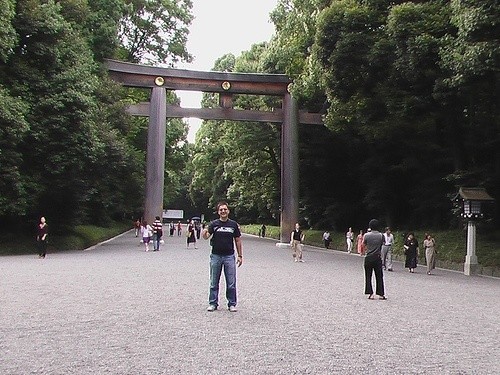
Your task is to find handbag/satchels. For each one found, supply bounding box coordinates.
[148,230,153,236]
[157,229,162,237]
[367,248,381,261]
[352,240,354,242]
[185,231,192,237]
[210,237,214,246]
[404,247,409,255]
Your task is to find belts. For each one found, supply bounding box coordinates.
[385,245,391,246]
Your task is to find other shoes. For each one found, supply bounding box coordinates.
[154,250,156,251]
[379,297,387,300]
[388,268,392,271]
[427,272,431,275]
[382,266,386,270]
[408,270,411,273]
[299,259,305,263]
[411,271,414,273]
[157,250,160,251]
[367,295,374,300]
[294,259,298,262]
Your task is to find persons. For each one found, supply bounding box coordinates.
[196,222,201,239]
[289,222,305,262]
[135,218,140,237]
[185,219,198,250]
[356,230,366,256]
[381,226,395,271]
[151,216,163,251]
[140,221,154,252]
[259,229,261,238]
[363,218,388,300]
[403,233,420,274]
[346,227,354,253]
[321,229,332,248]
[169,220,174,236]
[36,216,49,258]
[202,220,206,229]
[423,233,437,275]
[262,224,266,238]
[176,220,182,236]
[202,201,243,312]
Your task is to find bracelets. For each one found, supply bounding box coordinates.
[238,256,242,257]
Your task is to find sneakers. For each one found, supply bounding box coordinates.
[229,306,237,312]
[207,304,217,311]
